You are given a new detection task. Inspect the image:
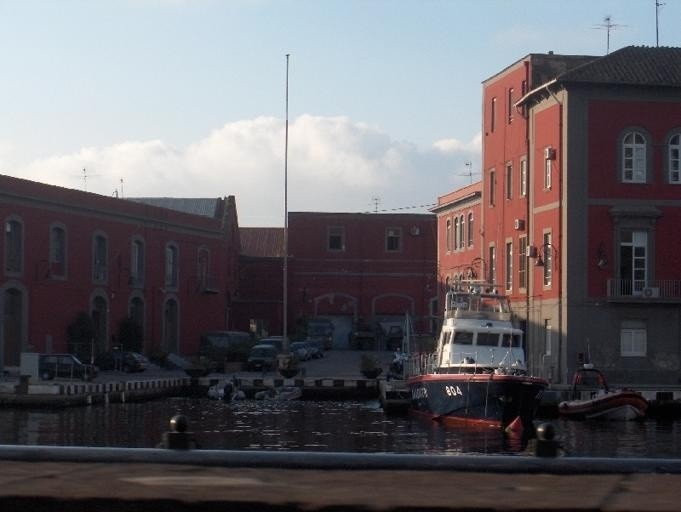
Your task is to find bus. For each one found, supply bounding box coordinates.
[297,317,334,351]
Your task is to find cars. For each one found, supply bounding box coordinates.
[246,335,324,372]
[96,351,149,372]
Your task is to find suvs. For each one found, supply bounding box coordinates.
[39,352,97,383]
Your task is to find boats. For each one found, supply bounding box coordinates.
[556,364,648,422]
[252,385,303,402]
[403,273,550,433]
[207,380,246,401]
